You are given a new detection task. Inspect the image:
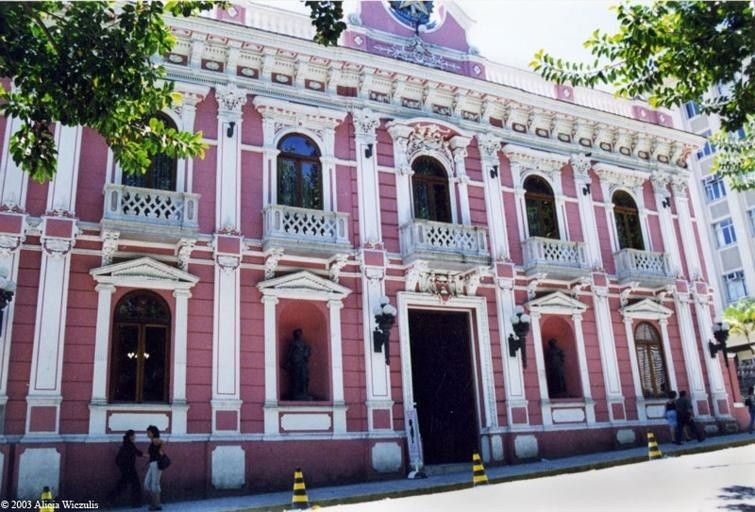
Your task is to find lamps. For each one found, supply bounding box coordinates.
[708,319,732,369]
[372,293,400,365]
[508,303,533,369]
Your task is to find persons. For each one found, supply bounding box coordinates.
[664,391,705,445]
[283,329,311,396]
[107,426,170,511]
[546,337,568,394]
[745,386,755,434]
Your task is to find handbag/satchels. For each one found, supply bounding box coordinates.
[157,454,171,470]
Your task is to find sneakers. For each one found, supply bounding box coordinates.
[148,504,162,510]
